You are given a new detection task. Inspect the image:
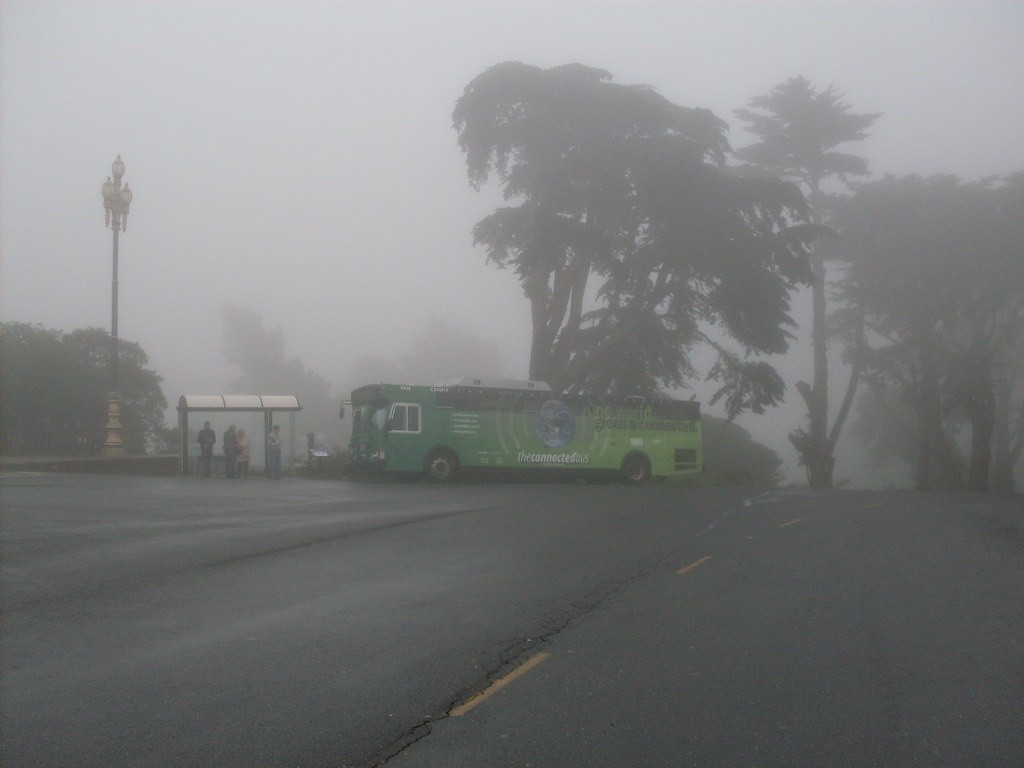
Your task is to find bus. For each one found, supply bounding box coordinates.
[338,382,706,485]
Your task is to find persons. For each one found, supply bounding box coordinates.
[222,423,239,477]
[267,425,285,479]
[234,429,250,477]
[198,421,216,477]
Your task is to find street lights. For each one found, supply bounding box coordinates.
[98,154,135,458]
